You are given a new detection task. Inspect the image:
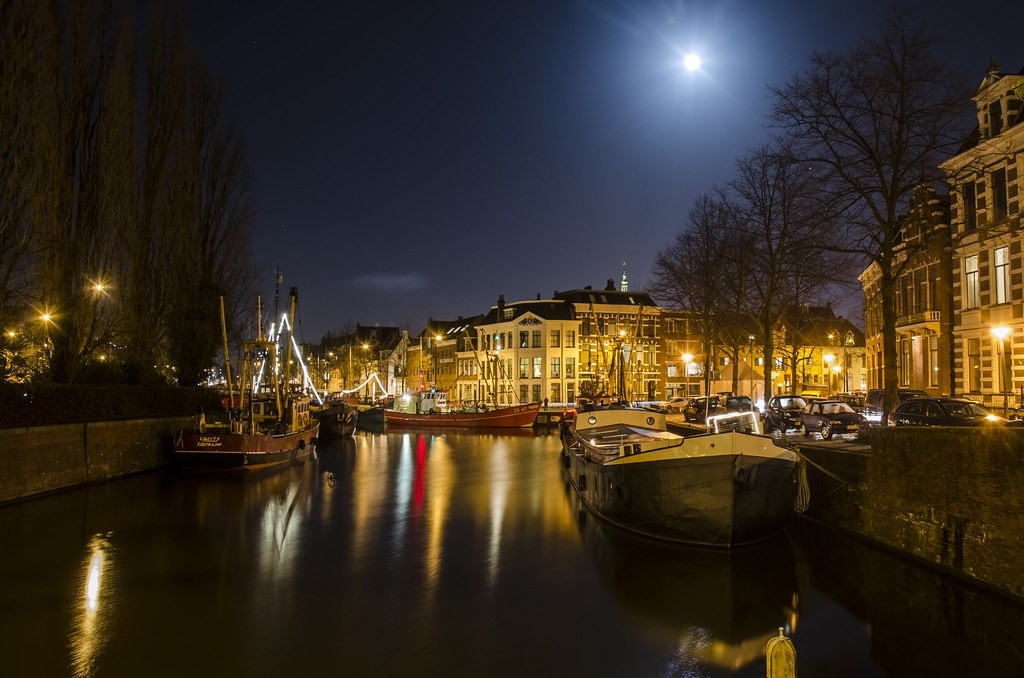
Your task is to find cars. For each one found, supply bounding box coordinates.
[802,392,866,420]
[764,395,809,434]
[799,400,868,441]
[715,395,759,416]
[658,397,689,413]
[684,395,721,425]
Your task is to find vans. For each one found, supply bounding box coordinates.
[865,388,931,426]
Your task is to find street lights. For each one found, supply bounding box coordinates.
[434,334,441,388]
[992,324,1011,420]
[680,352,694,402]
[363,343,369,402]
[614,336,625,402]
[747,332,756,405]
[830,328,855,393]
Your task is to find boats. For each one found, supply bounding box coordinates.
[554,400,804,551]
[314,399,360,438]
[383,395,543,429]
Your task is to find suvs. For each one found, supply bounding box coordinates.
[889,397,1010,427]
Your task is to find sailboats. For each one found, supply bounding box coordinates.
[163,287,322,475]
